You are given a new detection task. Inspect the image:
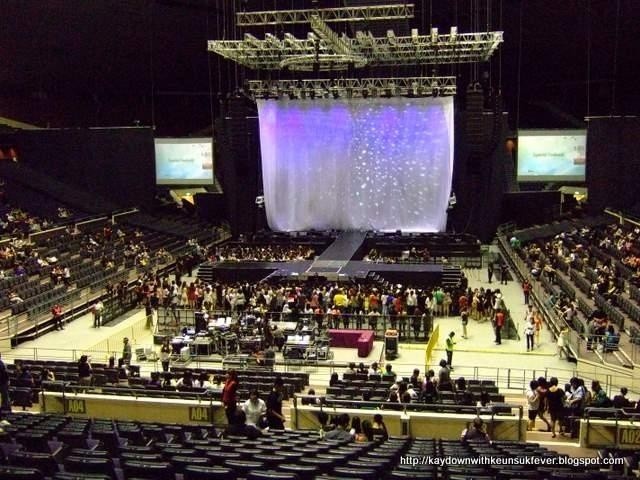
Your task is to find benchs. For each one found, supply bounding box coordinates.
[0,159,640,480]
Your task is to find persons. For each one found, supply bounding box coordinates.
[0,206,639,444]
